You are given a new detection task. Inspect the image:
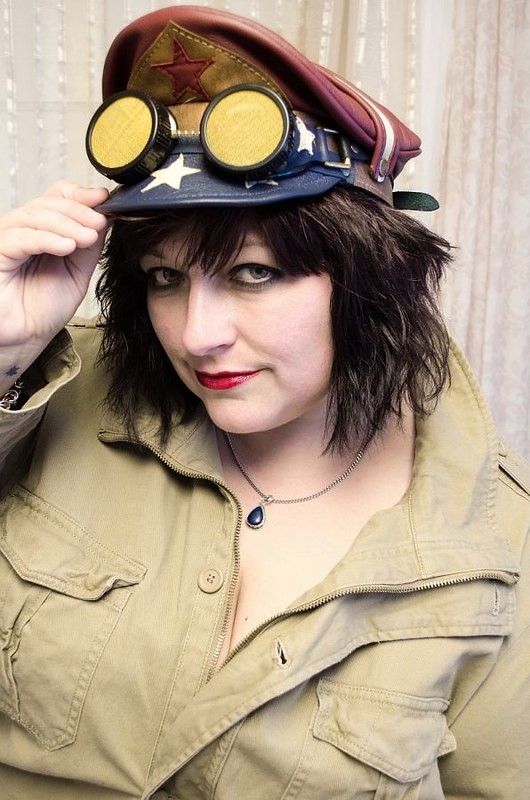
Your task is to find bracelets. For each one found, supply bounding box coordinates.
[0,378,26,413]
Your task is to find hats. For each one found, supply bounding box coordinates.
[91,6,440,211]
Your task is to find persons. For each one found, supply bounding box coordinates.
[0,5,530,800]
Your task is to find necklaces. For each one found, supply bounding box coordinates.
[225,420,375,530]
